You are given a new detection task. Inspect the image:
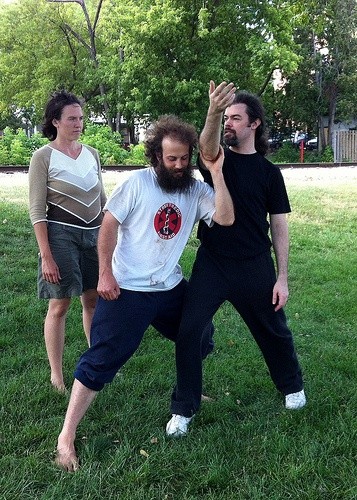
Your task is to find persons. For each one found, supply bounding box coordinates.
[28,93,108,391]
[166,80,306,438]
[56,116,234,471]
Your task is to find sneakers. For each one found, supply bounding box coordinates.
[166,413,195,438]
[284,388,306,410]
